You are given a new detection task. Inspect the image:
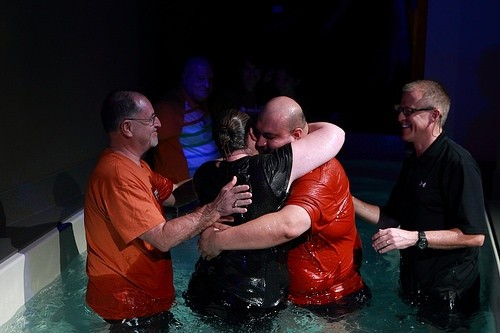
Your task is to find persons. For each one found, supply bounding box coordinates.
[151,56,221,216]
[186,106,346,329]
[196,95,372,318]
[351,79,489,330]
[238,57,302,107]
[85,88,252,333]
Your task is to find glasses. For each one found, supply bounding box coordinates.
[395,106,434,116]
[120,112,157,127]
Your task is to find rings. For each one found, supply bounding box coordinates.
[234,200,239,207]
[386,240,390,245]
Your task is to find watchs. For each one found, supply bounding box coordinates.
[417,231,428,250]
